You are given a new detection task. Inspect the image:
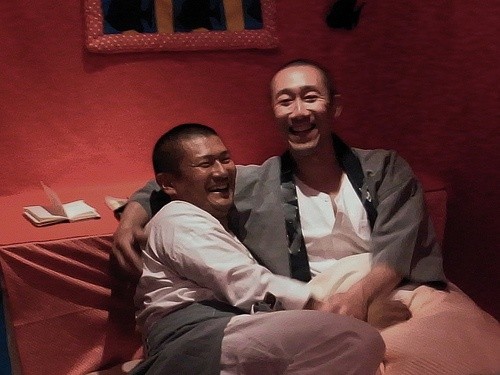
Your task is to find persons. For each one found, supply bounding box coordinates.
[109,57,500,375]
[134,123,412,375]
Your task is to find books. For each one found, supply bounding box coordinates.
[21,179,102,227]
